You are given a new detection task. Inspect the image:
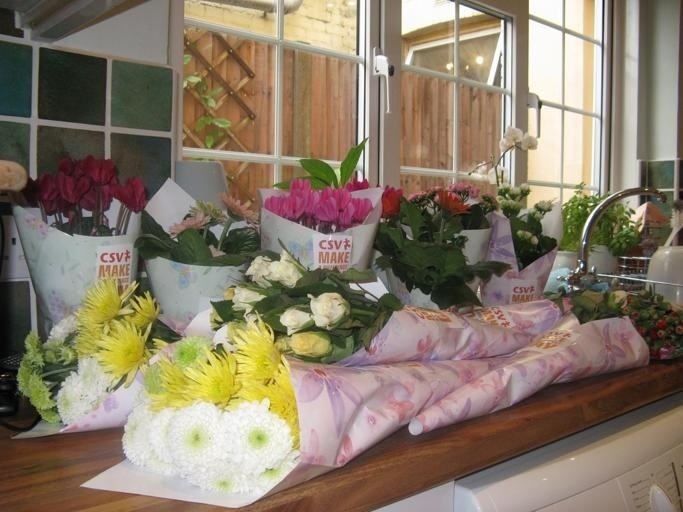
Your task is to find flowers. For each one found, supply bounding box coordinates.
[15,123,682,501]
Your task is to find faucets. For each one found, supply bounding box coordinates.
[579,187,667,259]
